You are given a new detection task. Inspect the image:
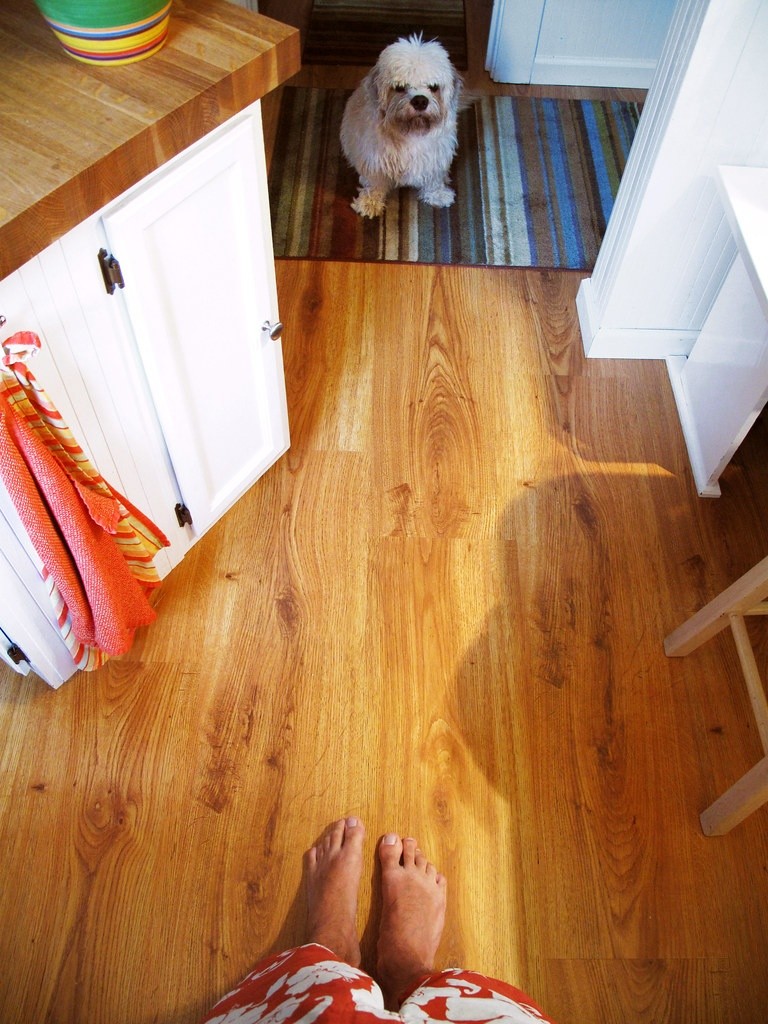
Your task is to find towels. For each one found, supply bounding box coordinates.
[0,333,169,672]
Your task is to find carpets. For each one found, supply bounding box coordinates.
[269,86,644,271]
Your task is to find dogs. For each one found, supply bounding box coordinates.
[340,30,464,219]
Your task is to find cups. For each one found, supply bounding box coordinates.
[33,0,173,64]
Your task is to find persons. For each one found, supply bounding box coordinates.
[198,815,564,1024]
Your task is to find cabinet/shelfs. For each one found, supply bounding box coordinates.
[0,97,292,692]
